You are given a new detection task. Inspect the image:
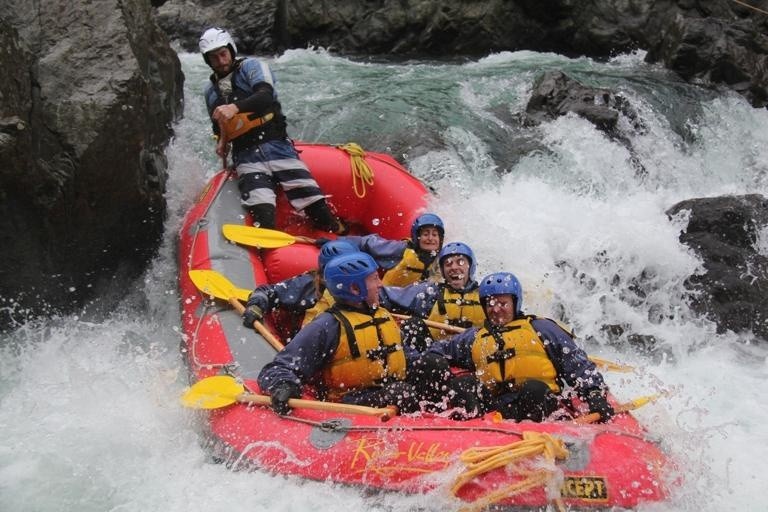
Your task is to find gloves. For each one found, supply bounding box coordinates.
[588,393,614,422]
[271,381,300,415]
[243,305,263,331]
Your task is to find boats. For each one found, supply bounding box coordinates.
[170,140,685,509]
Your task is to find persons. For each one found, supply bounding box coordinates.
[199,28,349,236]
[256,252,426,419]
[379,242,488,342]
[243,239,360,329]
[306,213,445,287]
[417,272,614,424]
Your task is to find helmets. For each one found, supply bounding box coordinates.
[319,214,521,315]
[199,27,237,65]
